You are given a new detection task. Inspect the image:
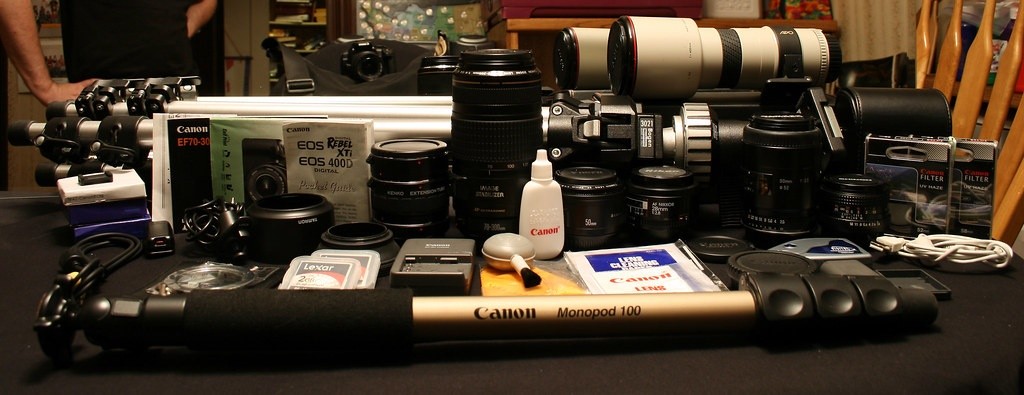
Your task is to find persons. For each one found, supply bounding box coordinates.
[56,0,227,98]
[31,0,68,78]
[0,1,102,109]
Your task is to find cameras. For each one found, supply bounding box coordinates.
[341,40,397,85]
